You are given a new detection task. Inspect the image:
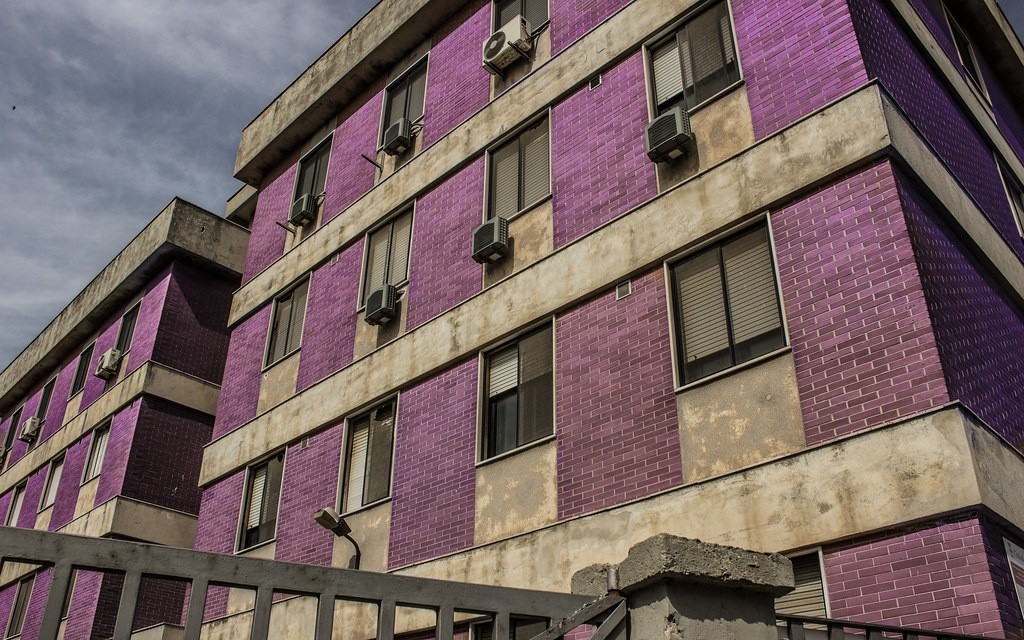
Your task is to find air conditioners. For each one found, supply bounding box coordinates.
[0,447,5,466]
[645,107,689,153]
[96,348,120,380]
[482,14,532,74]
[383,118,411,154]
[18,416,39,440]
[471,217,508,261]
[363,286,397,322]
[290,194,315,226]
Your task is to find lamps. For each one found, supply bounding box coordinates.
[314,507,361,570]
